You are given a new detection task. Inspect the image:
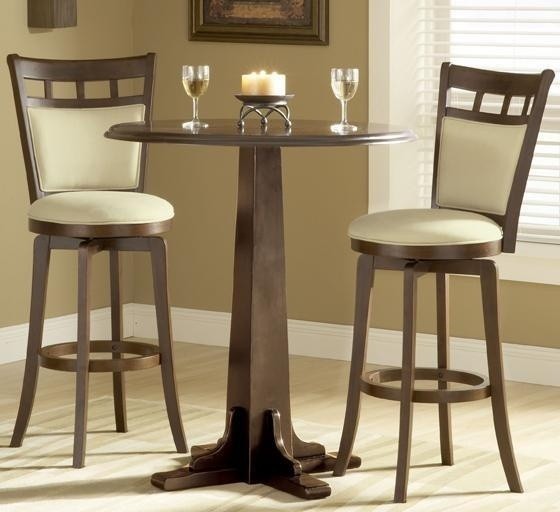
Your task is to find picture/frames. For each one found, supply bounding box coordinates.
[186,0,331,46]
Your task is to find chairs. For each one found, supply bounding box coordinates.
[333,60,555,502]
[7,52,188,469]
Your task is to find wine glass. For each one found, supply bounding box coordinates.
[329,67,360,134]
[180,64,211,130]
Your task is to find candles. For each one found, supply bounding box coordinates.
[241,69,286,96]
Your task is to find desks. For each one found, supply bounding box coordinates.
[103,117,411,500]
